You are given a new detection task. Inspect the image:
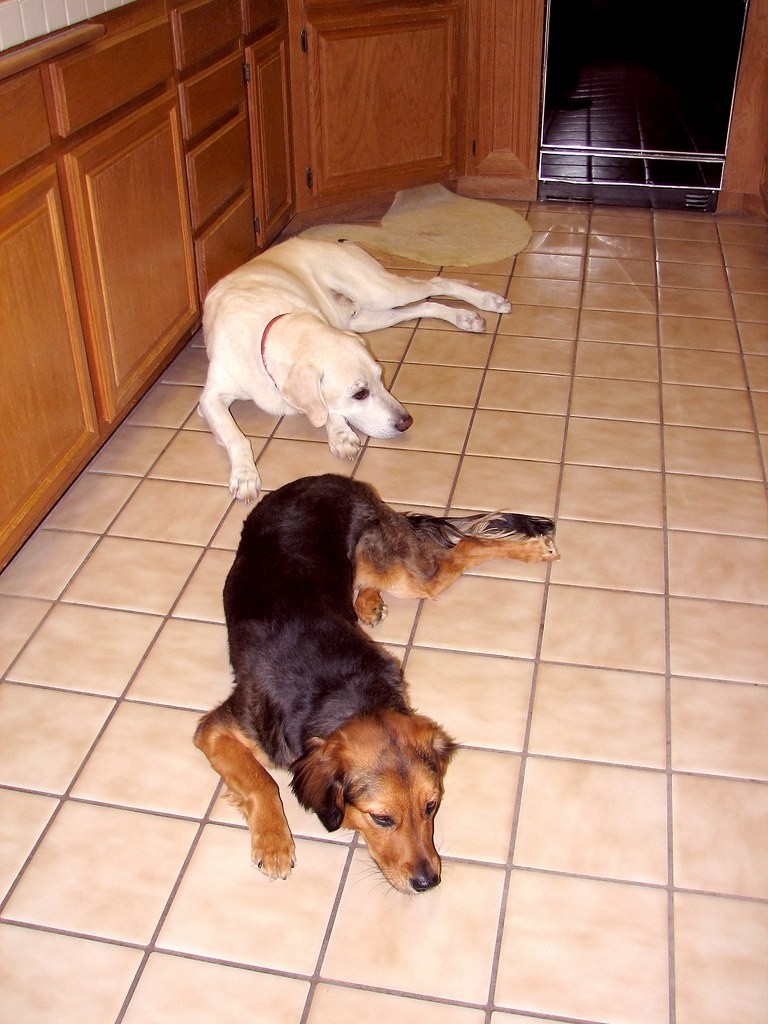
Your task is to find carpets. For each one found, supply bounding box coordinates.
[378,182,537,268]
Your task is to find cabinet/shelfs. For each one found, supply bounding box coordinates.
[0,0,546,578]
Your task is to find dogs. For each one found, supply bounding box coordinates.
[192,472,561,894]
[199,238,512,504]
